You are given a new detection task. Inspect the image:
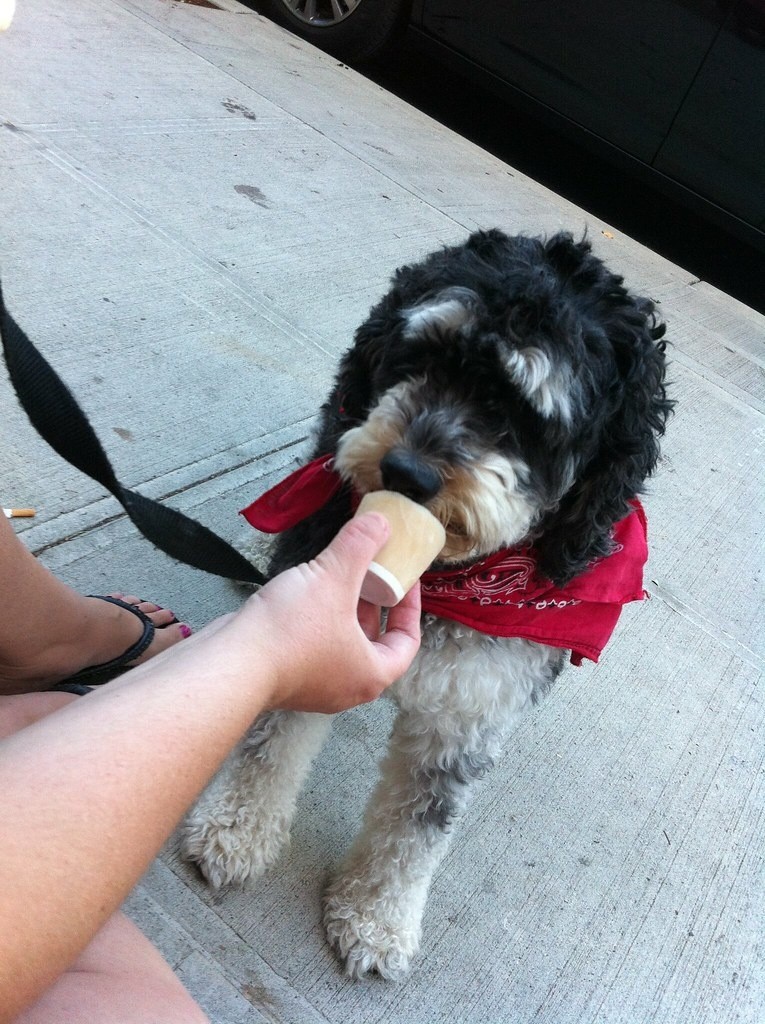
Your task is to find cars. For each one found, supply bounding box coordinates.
[239,0,765,262]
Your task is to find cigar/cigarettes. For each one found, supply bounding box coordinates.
[3,509,34,518]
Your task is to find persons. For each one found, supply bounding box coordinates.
[0,501,422,1024]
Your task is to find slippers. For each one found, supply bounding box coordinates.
[46,594,181,686]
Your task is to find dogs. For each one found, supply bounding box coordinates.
[174,218,680,984]
